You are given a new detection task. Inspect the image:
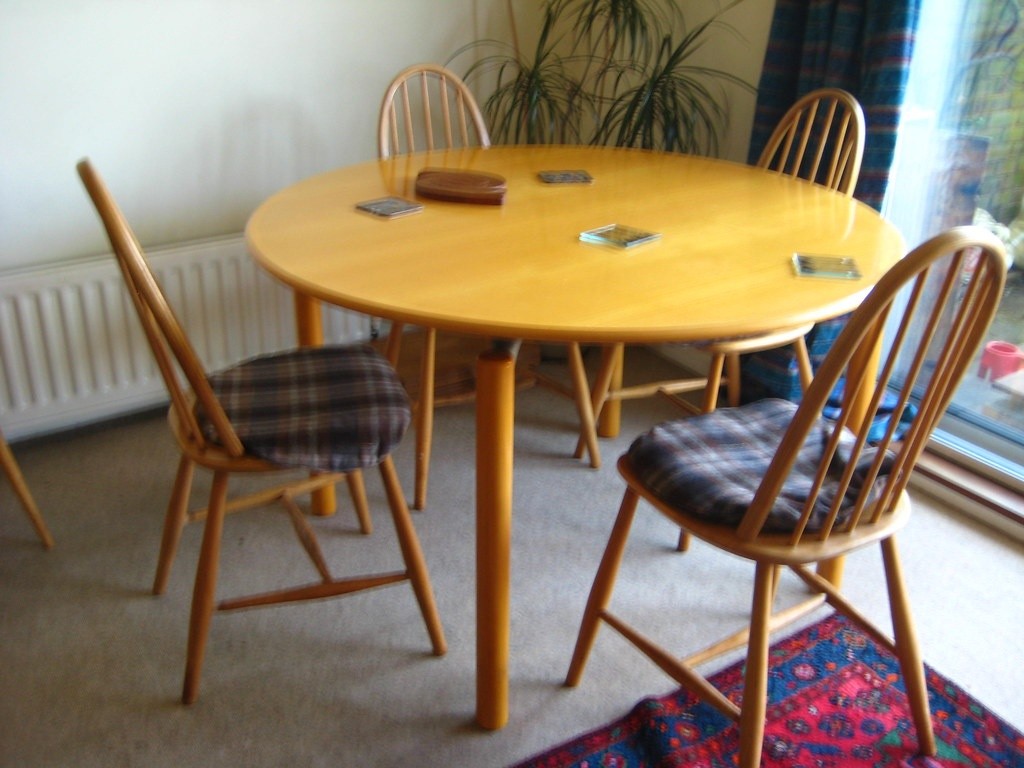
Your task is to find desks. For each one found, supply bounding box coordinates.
[246,143,907,730]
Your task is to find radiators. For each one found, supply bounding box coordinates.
[0,233,392,446]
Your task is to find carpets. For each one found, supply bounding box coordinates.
[503,610,1024,768]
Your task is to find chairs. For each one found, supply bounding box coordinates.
[563,226,1007,768]
[76,159,446,705]
[376,64,601,512]
[574,89,867,460]
